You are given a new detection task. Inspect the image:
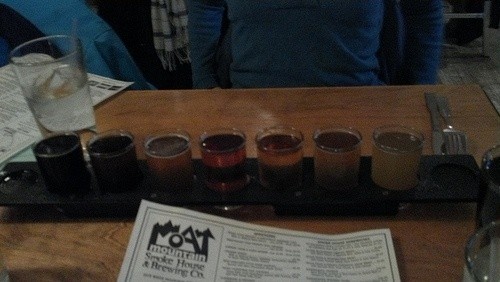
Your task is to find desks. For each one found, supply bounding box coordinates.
[0,85,500,282]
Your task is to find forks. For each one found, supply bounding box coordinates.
[435,95,467,157]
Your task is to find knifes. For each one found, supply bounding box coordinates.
[424,91,444,156]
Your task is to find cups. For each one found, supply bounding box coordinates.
[85,128,143,195]
[31,130,91,218]
[256,124,304,191]
[9,33,99,168]
[312,127,364,193]
[370,124,425,210]
[199,129,249,211]
[462,144,500,282]
[144,133,195,193]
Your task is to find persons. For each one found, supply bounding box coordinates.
[183,0,445,90]
